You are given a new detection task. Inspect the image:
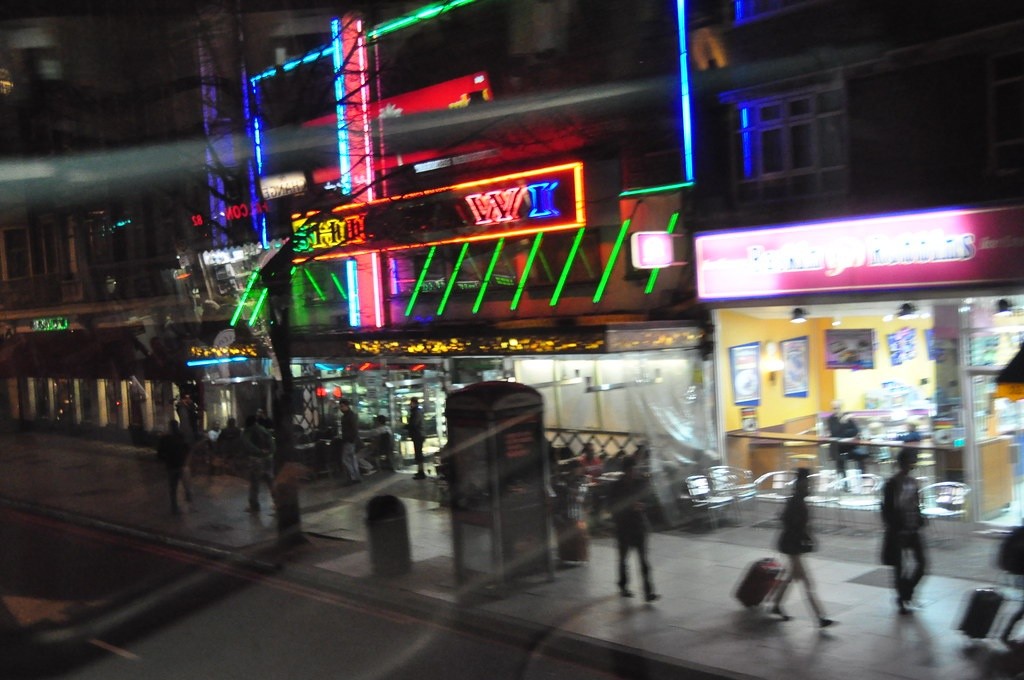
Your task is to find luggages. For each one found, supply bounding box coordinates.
[734,551,789,609]
[955,587,1003,639]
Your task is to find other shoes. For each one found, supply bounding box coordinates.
[646,593,659,601]
[897,596,914,613]
[819,617,832,628]
[621,589,632,596]
[772,605,789,620]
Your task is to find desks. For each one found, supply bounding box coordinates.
[293,434,366,464]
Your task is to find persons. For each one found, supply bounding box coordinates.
[241,413,276,507]
[161,420,193,511]
[575,444,606,515]
[610,457,661,602]
[770,469,833,628]
[338,400,364,485]
[889,417,921,476]
[208,417,245,452]
[356,414,392,477]
[401,397,428,480]
[882,447,926,614]
[827,400,870,493]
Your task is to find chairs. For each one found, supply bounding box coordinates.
[830,472,886,539]
[684,474,743,533]
[917,481,972,550]
[201,419,391,465]
[549,443,649,495]
[795,471,840,535]
[704,467,754,526]
[752,467,804,528]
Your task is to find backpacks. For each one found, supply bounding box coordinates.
[998,525,1024,575]
